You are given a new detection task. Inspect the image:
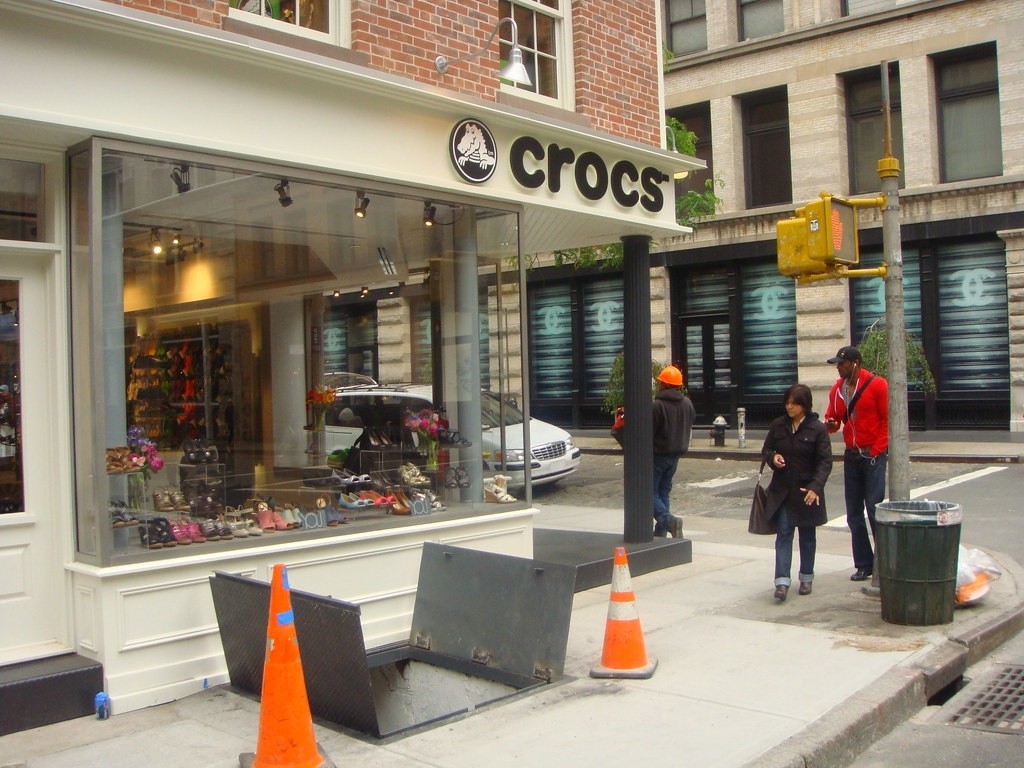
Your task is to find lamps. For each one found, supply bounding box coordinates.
[2,300,19,327]
[422,202,436,225]
[274,181,298,207]
[170,164,192,193]
[149,227,204,267]
[335,287,369,297]
[435,18,533,85]
[354,190,370,217]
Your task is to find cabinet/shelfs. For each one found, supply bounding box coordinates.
[107,471,150,551]
[357,448,430,489]
[181,464,228,519]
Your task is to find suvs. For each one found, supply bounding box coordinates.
[324,371,580,498]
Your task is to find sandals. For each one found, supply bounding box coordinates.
[107,426,472,550]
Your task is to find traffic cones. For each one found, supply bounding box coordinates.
[590,546,658,678]
[239,565,337,768]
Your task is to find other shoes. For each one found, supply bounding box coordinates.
[670,518,684,539]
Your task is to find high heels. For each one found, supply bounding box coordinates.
[494,475,507,493]
[486,485,518,503]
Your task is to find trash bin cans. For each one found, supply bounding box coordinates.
[874,501,962,627]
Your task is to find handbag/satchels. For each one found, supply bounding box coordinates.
[748,449,778,535]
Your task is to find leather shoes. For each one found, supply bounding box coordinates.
[799,581,813,594]
[851,570,871,581]
[774,585,788,601]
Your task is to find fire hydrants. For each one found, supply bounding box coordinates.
[709,415,727,446]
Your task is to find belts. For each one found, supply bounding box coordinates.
[850,448,869,453]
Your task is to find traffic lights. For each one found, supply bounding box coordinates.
[805,196,859,265]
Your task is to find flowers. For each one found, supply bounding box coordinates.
[307,384,338,412]
[129,426,164,510]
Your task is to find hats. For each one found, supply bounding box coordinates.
[827,347,863,364]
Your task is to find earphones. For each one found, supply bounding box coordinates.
[854,363,856,367]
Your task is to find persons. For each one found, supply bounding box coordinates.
[652,366,695,538]
[762,385,832,599]
[825,346,887,581]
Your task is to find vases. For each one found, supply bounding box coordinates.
[314,409,325,430]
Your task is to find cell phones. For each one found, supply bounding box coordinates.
[828,419,834,423]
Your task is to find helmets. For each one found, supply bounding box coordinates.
[657,366,683,386]
